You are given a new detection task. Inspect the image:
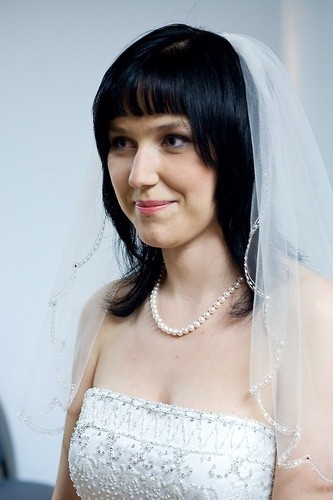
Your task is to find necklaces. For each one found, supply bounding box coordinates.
[149,240,252,337]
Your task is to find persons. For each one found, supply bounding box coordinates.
[42,22,331,499]
